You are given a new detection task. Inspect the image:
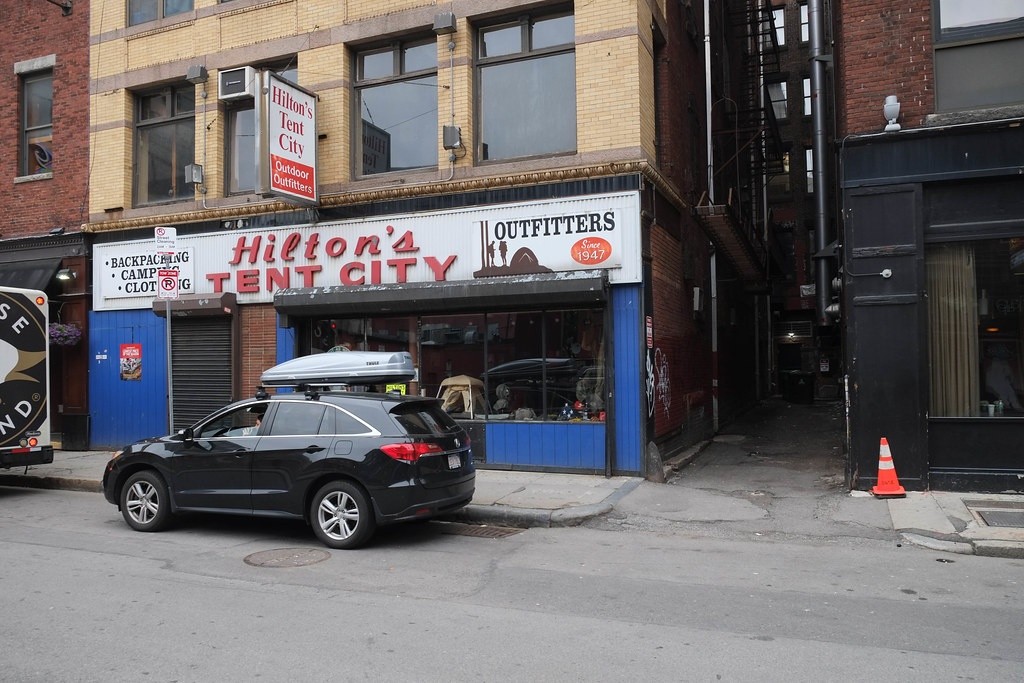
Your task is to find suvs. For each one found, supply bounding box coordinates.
[449,386,586,421]
[103,382,477,549]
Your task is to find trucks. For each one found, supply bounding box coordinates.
[0,287,53,467]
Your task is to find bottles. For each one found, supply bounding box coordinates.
[583,403,589,421]
[999,400,1003,405]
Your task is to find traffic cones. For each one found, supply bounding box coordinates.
[871,437,906,499]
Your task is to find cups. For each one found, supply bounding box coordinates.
[988,404,995,416]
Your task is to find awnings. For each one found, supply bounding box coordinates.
[0,258,64,291]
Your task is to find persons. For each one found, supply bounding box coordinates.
[249,414,264,436]
[494,383,514,411]
